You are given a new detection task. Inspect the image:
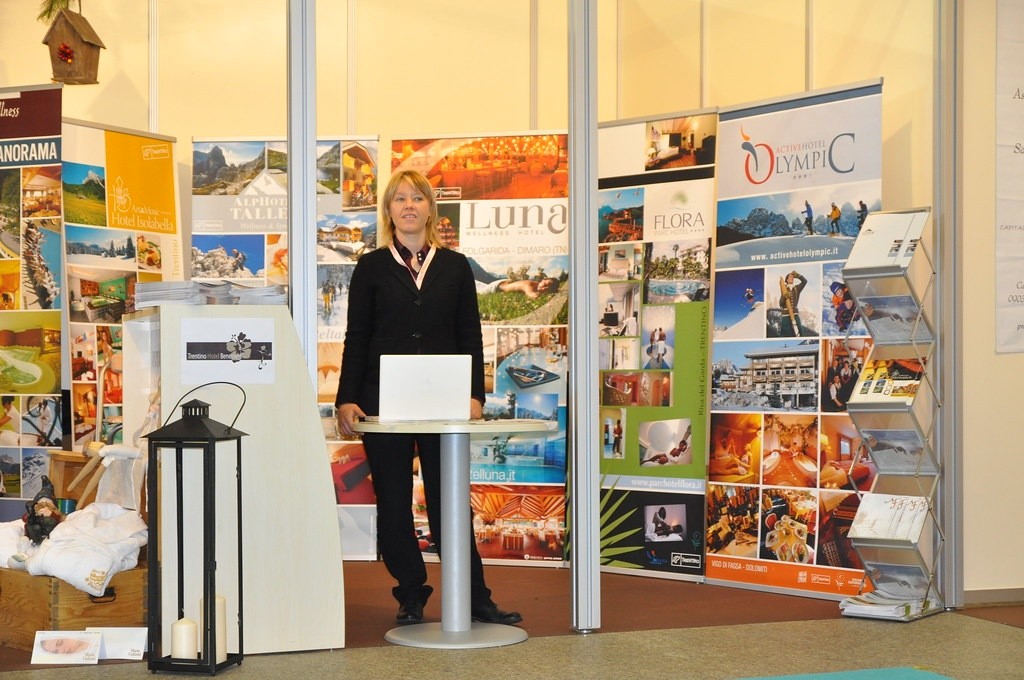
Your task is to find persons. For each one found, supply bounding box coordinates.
[710,428,753,477]
[826,202,842,234]
[100,331,115,363]
[641,439,687,466]
[321,278,344,312]
[36,399,52,443]
[856,200,868,230]
[474,277,562,298]
[335,170,523,628]
[825,357,862,409]
[779,270,808,336]
[23,219,60,308]
[612,419,623,453]
[800,199,815,235]
[231,248,247,273]
[743,287,754,301]
[0,396,21,432]
[830,281,860,333]
[789,442,801,458]
[650,327,666,361]
[652,507,683,536]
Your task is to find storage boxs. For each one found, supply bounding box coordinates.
[0,565,148,655]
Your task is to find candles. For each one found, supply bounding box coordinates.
[171,596,228,666]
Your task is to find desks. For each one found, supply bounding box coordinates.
[352,418,551,649]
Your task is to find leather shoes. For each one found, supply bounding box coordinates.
[471,600,523,624]
[396,600,424,624]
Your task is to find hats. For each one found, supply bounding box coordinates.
[830,282,842,294]
[843,291,852,301]
